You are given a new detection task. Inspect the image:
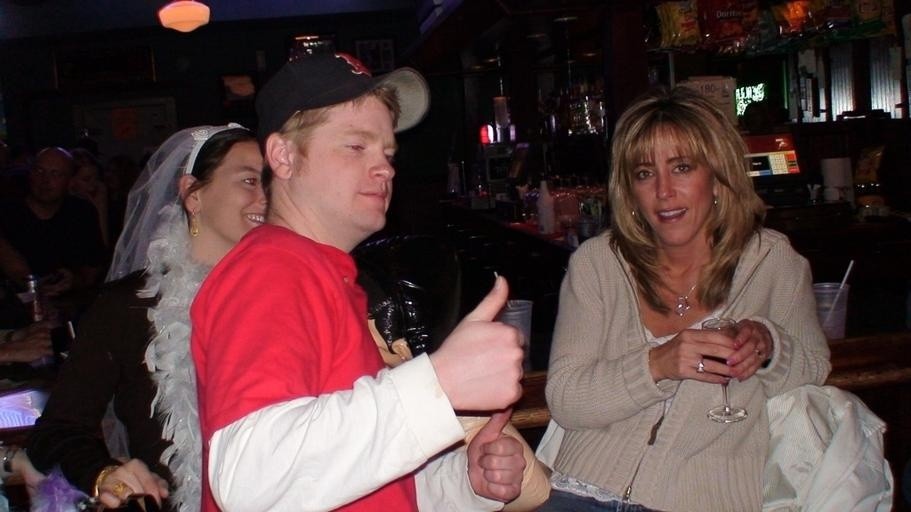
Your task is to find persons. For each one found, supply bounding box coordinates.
[28,126,269,510]
[2,144,125,332]
[533,83,833,510]
[188,45,528,511]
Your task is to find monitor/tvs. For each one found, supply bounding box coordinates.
[740,132,809,184]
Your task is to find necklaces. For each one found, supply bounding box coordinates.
[668,284,696,316]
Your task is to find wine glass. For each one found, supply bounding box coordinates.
[700,319,749,423]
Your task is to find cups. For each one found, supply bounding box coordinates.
[813,282,853,341]
[496,299,532,374]
[564,198,608,252]
[807,190,820,207]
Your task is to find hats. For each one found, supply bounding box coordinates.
[251,51,431,156]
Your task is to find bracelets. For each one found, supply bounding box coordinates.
[4,444,18,472]
[95,465,118,497]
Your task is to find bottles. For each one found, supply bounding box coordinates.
[538,181,557,235]
[565,76,606,135]
[473,174,489,200]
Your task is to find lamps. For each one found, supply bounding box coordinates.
[158,0,211,33]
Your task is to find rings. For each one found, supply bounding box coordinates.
[756,349,761,355]
[111,480,128,496]
[697,359,704,373]
